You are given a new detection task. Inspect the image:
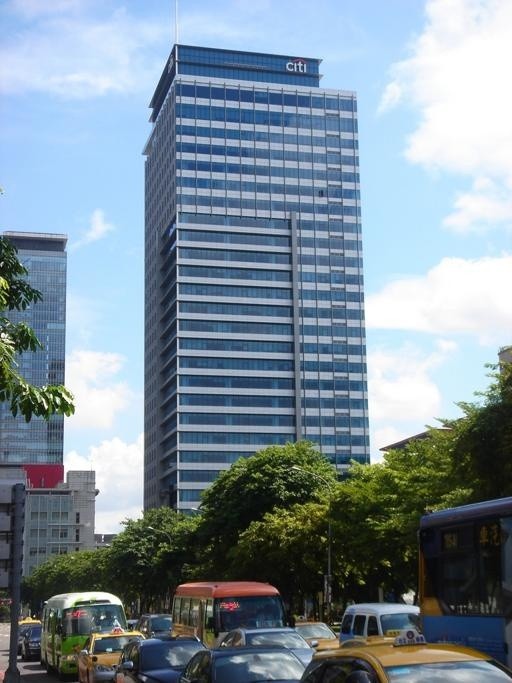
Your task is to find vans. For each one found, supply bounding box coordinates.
[339,600,420,645]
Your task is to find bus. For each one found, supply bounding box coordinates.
[41,586,126,674]
[165,571,288,646]
[415,495,510,656]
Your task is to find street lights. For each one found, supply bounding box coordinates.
[147,523,172,548]
[291,465,334,616]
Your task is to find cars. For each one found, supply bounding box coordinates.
[77,626,146,683]
[125,612,173,645]
[178,647,309,683]
[214,625,318,668]
[112,632,210,683]
[17,616,42,661]
[300,627,512,683]
[294,618,337,640]
